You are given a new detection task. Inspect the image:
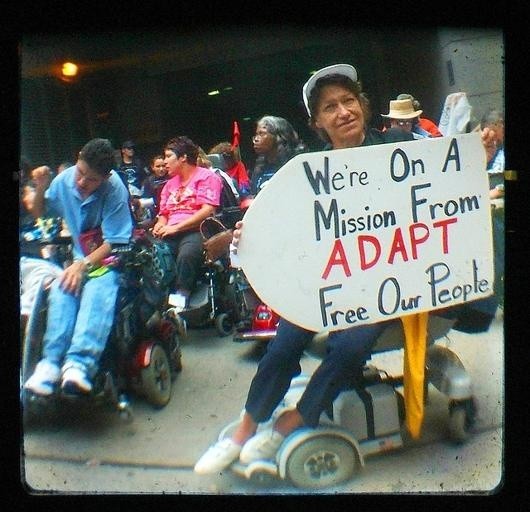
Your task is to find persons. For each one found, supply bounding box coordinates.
[18,92,504,399]
[191,61,500,480]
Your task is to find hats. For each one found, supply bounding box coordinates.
[301,63,358,117]
[122,140,135,148]
[380,99,423,120]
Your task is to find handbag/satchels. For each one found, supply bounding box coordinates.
[78,223,148,268]
[199,217,233,259]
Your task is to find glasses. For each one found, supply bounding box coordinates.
[395,120,414,126]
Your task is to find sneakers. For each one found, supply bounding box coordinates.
[238,408,291,466]
[168,292,186,314]
[193,438,240,476]
[23,358,61,396]
[61,361,93,395]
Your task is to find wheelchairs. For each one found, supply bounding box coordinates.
[22,170,245,418]
[218,311,481,489]
